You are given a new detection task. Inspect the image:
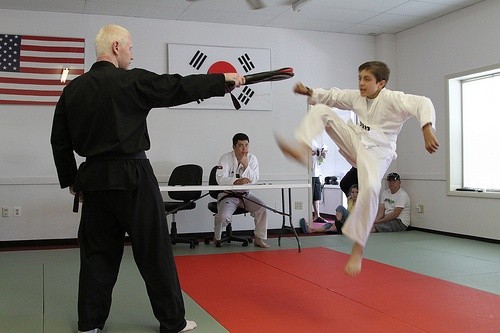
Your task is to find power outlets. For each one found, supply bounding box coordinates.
[14,207,21,216]
[418,205,423,213]
[2,207,9,217]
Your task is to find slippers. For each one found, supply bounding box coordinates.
[313,216,329,223]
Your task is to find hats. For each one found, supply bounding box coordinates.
[387,173,401,180]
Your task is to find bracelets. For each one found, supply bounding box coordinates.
[303,87,313,96]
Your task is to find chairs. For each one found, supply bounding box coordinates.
[208,166,253,247]
[163,164,203,249]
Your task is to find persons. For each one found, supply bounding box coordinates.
[215,133,271,248]
[50,24,246,333]
[371,173,411,232]
[277,61,440,277]
[311,139,329,223]
[300,184,359,234]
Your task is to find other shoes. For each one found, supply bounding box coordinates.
[300,217,309,235]
[335,219,345,235]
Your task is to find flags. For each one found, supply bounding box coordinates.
[0,34,85,105]
[168,44,273,111]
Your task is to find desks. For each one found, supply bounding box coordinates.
[159,184,312,252]
[322,185,343,206]
